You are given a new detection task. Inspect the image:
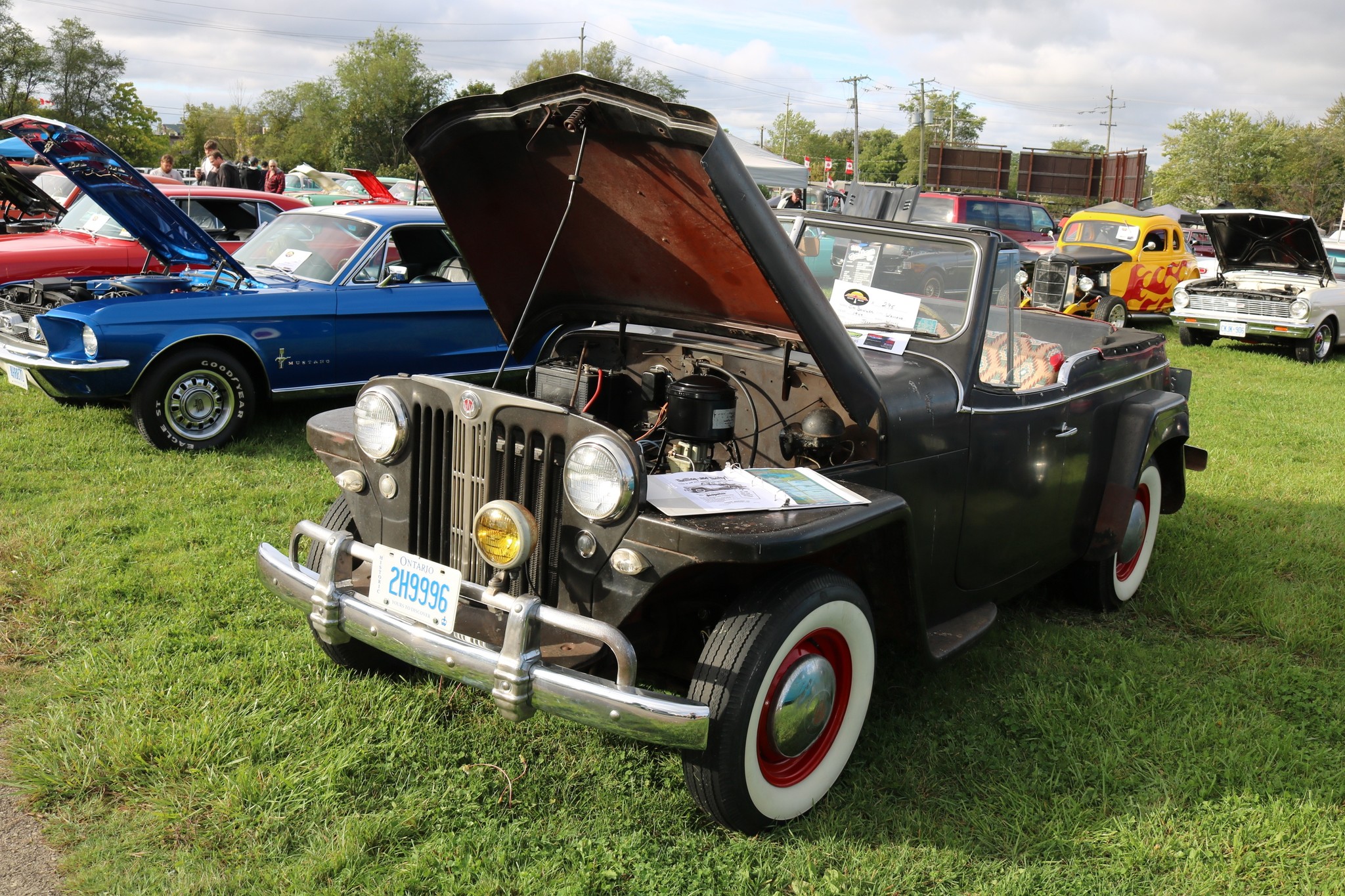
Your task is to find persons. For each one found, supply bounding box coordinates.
[31,154,47,165]
[777,188,804,210]
[194,140,287,193]
[149,154,186,183]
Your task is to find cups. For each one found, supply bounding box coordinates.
[195,167,201,175]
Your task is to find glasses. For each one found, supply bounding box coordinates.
[270,165,275,167]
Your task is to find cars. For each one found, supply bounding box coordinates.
[767,187,1345,366]
[0,115,532,454]
[252,75,1218,838]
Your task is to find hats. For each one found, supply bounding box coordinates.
[793,189,802,198]
[250,157,261,163]
[261,161,268,166]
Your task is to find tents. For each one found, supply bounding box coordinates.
[724,130,809,210]
[0,136,46,159]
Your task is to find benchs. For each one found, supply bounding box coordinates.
[935,323,1063,391]
